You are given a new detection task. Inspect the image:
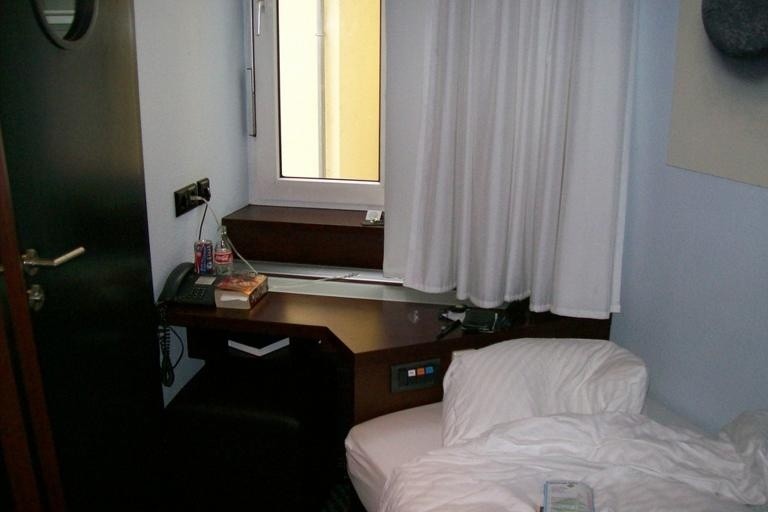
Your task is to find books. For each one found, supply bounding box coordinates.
[214,274,269,312]
[543,479,594,512]
[228,324,291,356]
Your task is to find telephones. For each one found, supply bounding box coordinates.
[158,263,225,305]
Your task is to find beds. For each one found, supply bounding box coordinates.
[343,395,767,512]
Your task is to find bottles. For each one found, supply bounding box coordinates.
[212,226,233,276]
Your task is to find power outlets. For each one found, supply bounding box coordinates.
[174,183,200,217]
[197,177,211,205]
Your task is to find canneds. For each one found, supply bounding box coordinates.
[194,239,213,274]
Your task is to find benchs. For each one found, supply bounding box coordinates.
[222,204,384,269]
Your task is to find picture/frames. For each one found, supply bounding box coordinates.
[700,0,768,61]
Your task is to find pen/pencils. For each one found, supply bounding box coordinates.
[437,319,462,339]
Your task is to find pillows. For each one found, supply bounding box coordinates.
[442,340,649,445]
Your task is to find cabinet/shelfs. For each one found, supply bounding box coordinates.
[156,291,614,424]
